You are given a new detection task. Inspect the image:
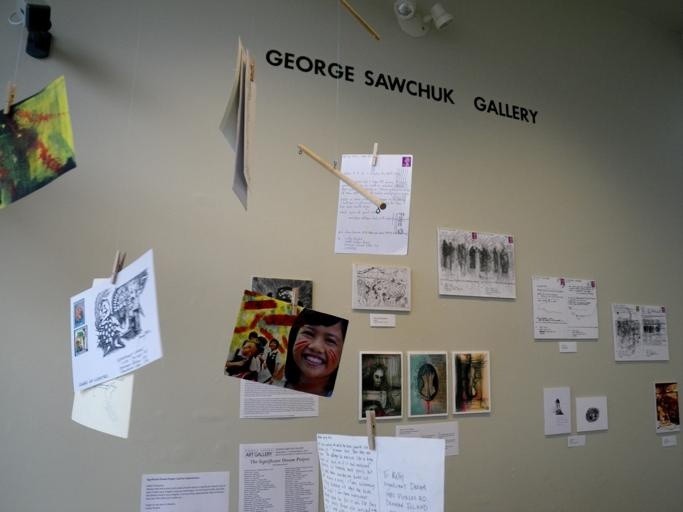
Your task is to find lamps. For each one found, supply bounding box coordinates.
[393,0,453,39]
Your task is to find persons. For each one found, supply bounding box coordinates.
[656,384,679,424]
[272,307,349,396]
[442,239,510,274]
[224,330,281,384]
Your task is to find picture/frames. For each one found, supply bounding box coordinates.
[576,395,609,432]
[359,351,403,420]
[543,387,571,435]
[407,351,448,418]
[653,381,682,433]
[451,350,492,415]
[352,263,412,311]
